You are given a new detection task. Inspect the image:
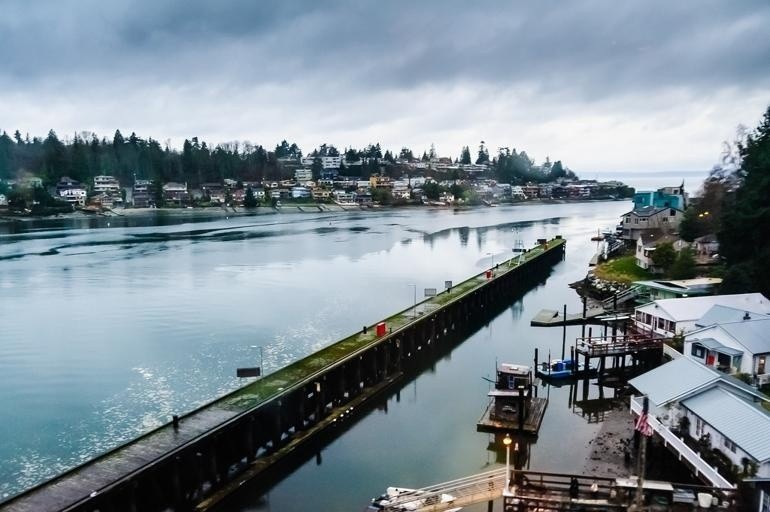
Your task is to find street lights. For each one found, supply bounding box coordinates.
[503,433,511,489]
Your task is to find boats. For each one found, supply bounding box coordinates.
[536,359,597,381]
[369,487,464,512]
